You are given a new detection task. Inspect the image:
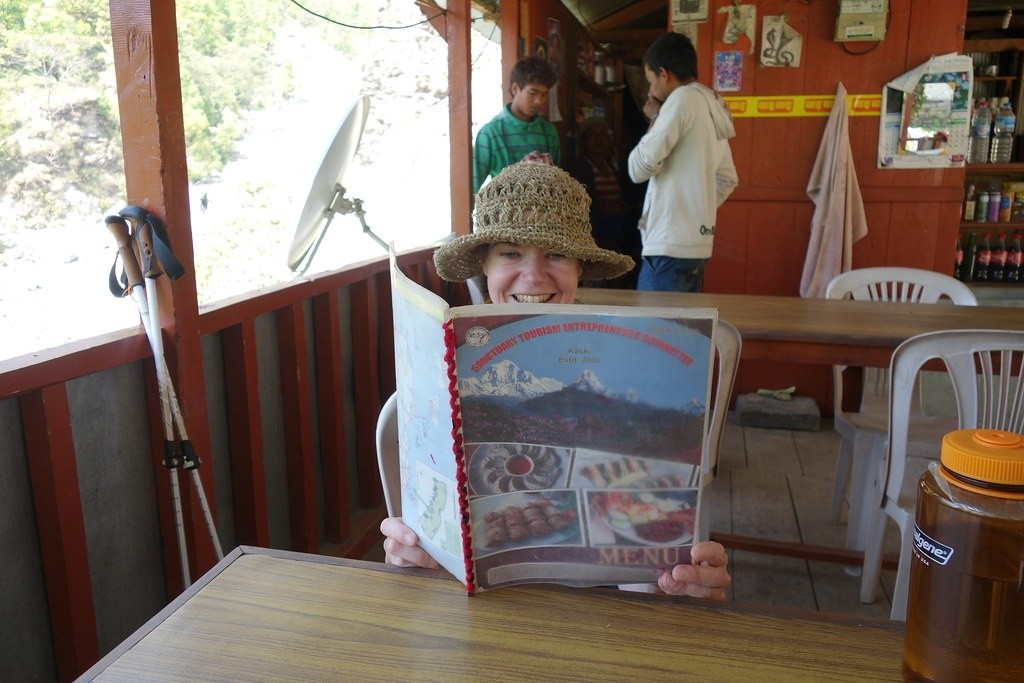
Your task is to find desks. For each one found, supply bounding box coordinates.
[71,545,909,683]
[573,287,1024,568]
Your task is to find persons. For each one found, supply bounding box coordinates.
[380,161,731,600]
[628,31,738,292]
[473,59,561,195]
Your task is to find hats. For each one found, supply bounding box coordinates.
[433,160,634,282]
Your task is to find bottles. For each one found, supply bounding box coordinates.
[990,103,1015,162]
[991,233,1006,281]
[899,428,1024,682]
[962,232,977,280]
[954,232,963,278]
[961,184,1024,224]
[966,102,991,163]
[973,233,991,280]
[1006,234,1023,282]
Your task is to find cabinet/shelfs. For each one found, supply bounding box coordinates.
[959,35,1024,301]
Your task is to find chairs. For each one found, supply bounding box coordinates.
[860,330,1024,622]
[375,389,655,593]
[825,266,981,576]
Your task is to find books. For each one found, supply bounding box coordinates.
[389,243,718,599]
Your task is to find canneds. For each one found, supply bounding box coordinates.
[975,190,1015,223]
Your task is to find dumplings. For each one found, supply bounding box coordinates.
[480,444,560,493]
